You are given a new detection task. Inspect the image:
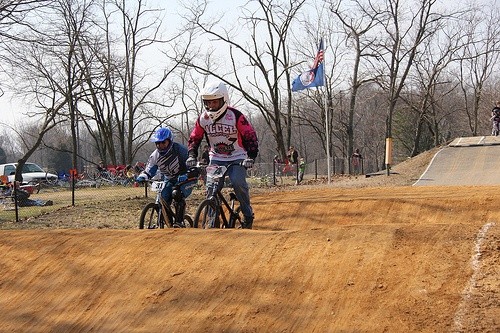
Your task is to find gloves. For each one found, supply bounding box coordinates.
[244,159,255,168]
[178,175,187,182]
[186,158,195,166]
[137,175,145,181]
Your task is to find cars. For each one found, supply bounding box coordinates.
[0,161,59,191]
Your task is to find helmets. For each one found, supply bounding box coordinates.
[200,82,229,120]
[155,128,173,156]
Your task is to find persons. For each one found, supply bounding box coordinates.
[95,161,107,174]
[352,149,363,159]
[12,181,53,207]
[295,157,305,185]
[135,127,199,228]
[491,101,500,134]
[186,82,259,230]
[127,165,135,178]
[287,144,298,170]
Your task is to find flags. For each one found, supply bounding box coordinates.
[292,37,326,92]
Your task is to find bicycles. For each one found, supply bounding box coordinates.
[133,177,194,229]
[261,156,284,187]
[490,114,500,136]
[27,165,133,194]
[187,161,255,230]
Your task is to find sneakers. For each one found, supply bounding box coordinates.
[244,216,253,228]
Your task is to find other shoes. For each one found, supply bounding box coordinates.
[173,223,183,228]
